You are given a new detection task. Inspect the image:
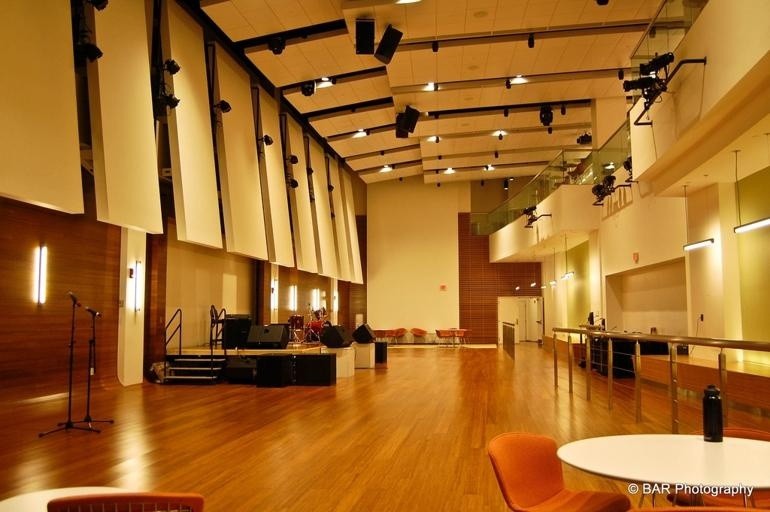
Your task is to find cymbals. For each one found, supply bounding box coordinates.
[317,315,328,318]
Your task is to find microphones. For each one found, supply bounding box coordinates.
[84,306,100,316]
[68,292,81,307]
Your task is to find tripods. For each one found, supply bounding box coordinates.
[57,315,114,430]
[289,321,302,343]
[38,301,100,437]
[303,312,320,342]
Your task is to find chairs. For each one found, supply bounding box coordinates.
[486,426,770,512]
[372,328,473,349]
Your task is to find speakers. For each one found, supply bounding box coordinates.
[352,324,376,343]
[295,352,337,386]
[246,325,288,349]
[375,341,387,363]
[320,326,354,348]
[221,318,251,349]
[256,354,292,388]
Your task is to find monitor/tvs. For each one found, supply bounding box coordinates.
[599,318,605,331]
[588,312,594,325]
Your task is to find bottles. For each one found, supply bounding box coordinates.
[702,383,723,442]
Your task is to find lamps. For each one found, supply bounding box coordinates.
[300,80,316,97]
[86,43,299,189]
[590,174,632,201]
[621,50,674,105]
[353,15,404,66]
[393,105,422,140]
[539,104,554,127]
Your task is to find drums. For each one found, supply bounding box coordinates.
[290,314,304,331]
[314,307,328,320]
[306,320,332,342]
